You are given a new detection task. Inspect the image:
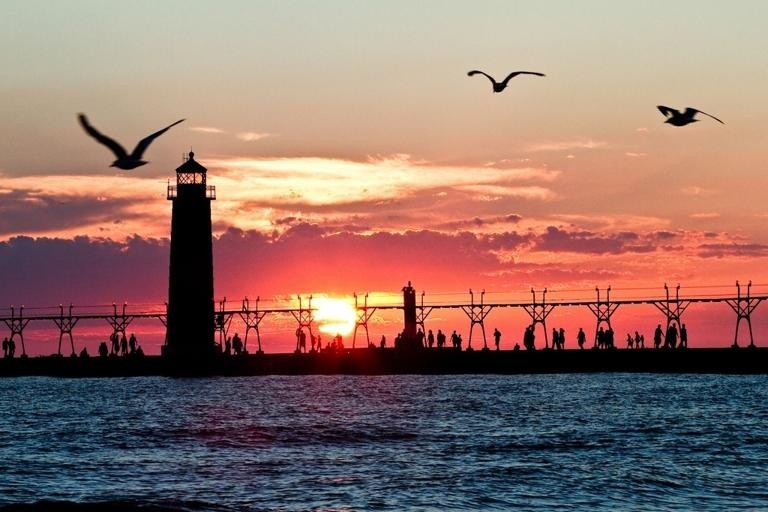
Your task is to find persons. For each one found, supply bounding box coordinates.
[78,330,144,359]
[1,337,9,357]
[235,337,244,354]
[379,333,388,347]
[231,332,239,353]
[417,319,687,350]
[298,327,345,358]
[8,338,16,358]
[226,337,231,354]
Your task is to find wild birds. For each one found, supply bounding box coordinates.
[467,70,545,93]
[78,114,185,171]
[657,104,725,126]
